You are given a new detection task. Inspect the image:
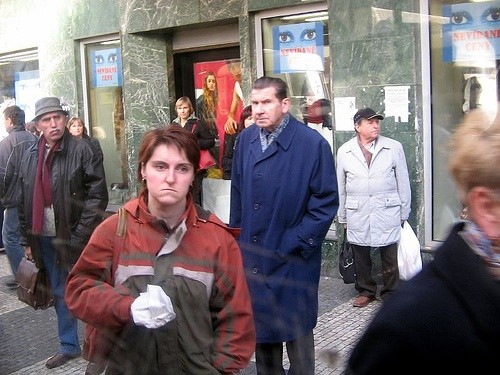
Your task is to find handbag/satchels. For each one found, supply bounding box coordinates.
[80,324,115,362]
[15,255,53,311]
[337,226,359,285]
[194,149,216,173]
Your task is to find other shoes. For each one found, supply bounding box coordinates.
[5,280,18,289]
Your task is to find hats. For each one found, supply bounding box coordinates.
[31,97,68,121]
[353,107,384,122]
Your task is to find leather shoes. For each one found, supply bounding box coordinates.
[353,296,371,307]
[46,350,81,368]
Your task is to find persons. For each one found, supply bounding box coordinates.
[344,102,500,375]
[16,97,109,375]
[223,59,244,156]
[196,72,220,169]
[66,117,104,163]
[336,108,412,308]
[172,96,215,206]
[0,106,38,289]
[64,125,256,375]
[222,105,254,180]
[229,77,340,375]
